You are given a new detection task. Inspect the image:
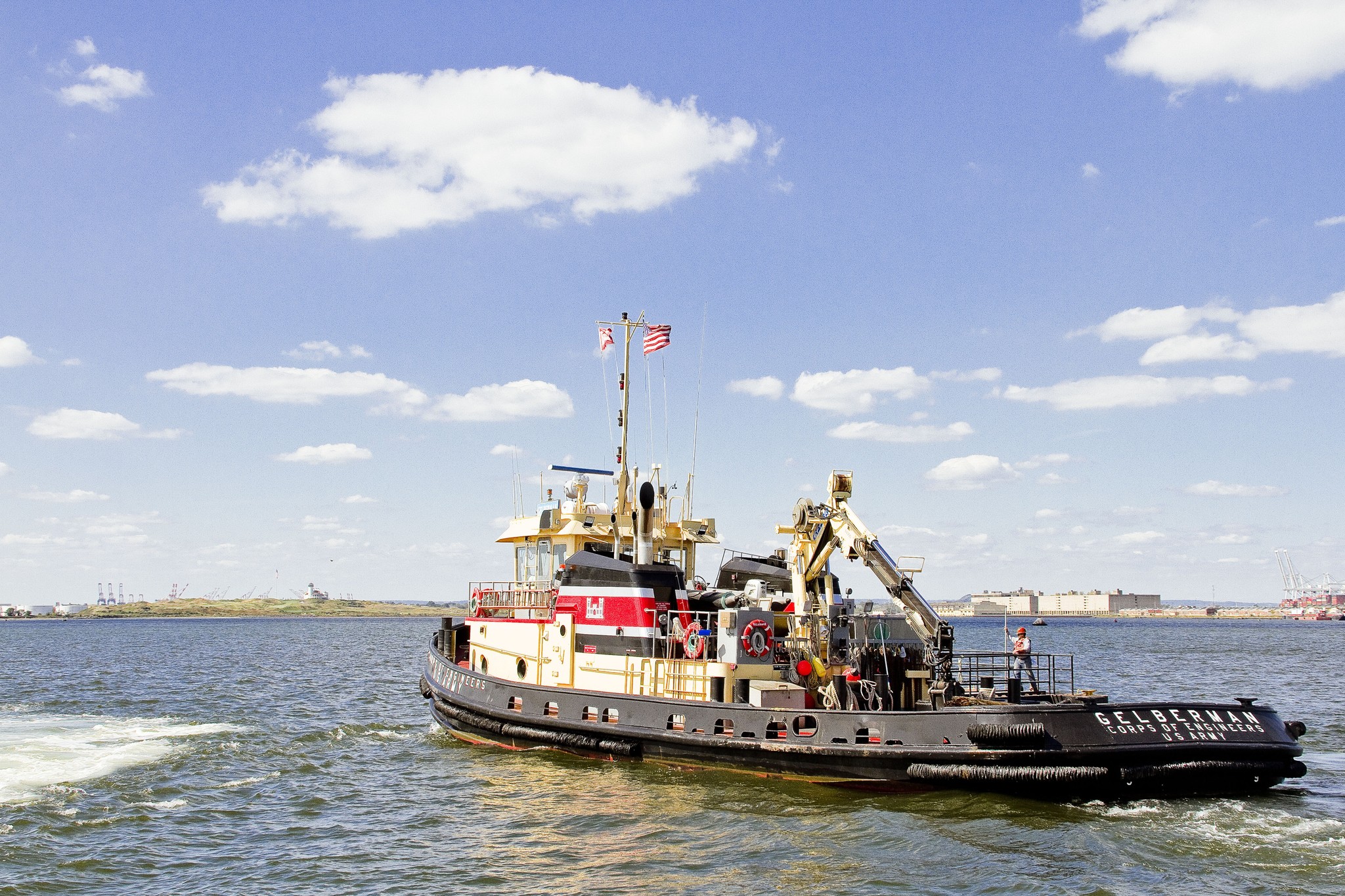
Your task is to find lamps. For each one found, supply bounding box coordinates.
[696,523,709,536]
[582,515,595,528]
[860,599,874,615]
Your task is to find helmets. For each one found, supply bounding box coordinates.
[1017,627,1027,634]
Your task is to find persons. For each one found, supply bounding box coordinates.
[1003,626,1041,695]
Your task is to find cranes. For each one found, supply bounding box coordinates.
[1274,550,1345,600]
[96,583,144,606]
[155,584,189,600]
[259,587,272,599]
[240,586,255,599]
[202,586,230,600]
[290,589,306,599]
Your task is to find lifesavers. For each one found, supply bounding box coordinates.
[743,619,773,657]
[683,622,704,659]
[472,587,499,618]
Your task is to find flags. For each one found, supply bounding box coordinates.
[642,322,672,356]
[598,327,615,351]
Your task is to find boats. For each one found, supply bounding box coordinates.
[1293,615,1344,622]
[417,122,1307,801]
